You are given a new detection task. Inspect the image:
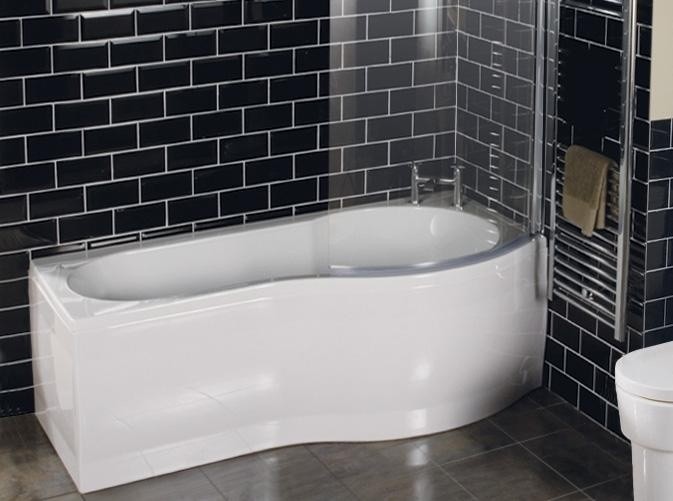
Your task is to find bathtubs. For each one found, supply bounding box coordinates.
[28,188,548,493]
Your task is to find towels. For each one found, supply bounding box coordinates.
[561,145,610,239]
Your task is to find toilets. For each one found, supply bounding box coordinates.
[615,341,673,501]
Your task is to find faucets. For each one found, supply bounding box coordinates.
[418,175,439,194]
